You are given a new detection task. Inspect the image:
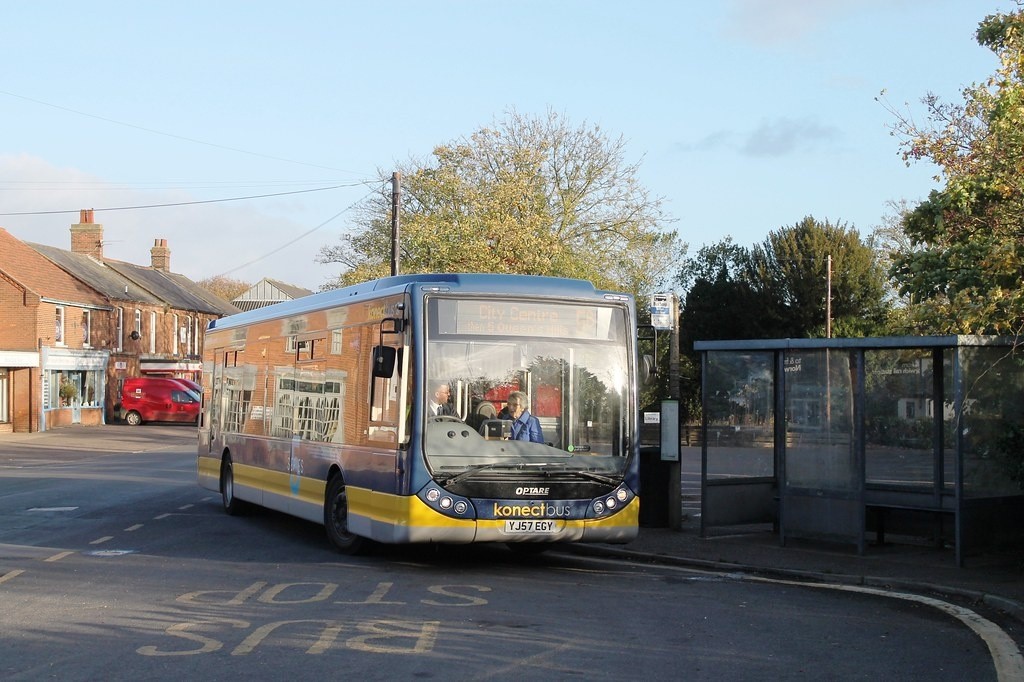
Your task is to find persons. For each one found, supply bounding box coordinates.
[427,380,461,422]
[498,391,545,444]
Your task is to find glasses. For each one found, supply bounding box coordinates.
[438,391,449,394]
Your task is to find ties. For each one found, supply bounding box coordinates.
[437,408,440,416]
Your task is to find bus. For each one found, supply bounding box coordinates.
[194,272,661,553]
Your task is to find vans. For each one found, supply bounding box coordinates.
[118,377,200,426]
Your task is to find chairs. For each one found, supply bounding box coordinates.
[475,401,498,427]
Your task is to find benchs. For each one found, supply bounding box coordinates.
[773,497,956,550]
